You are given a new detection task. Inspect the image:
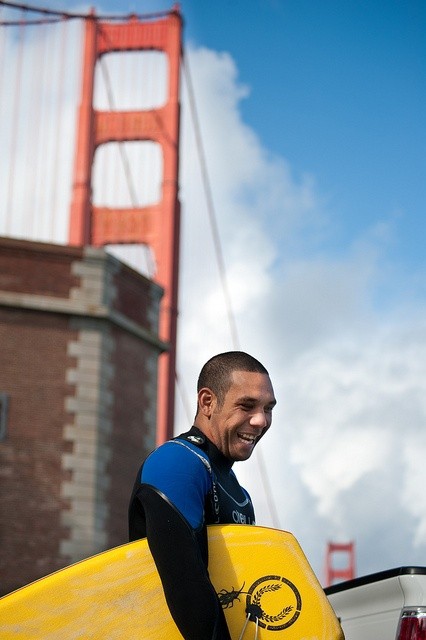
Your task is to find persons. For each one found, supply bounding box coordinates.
[128,351,277,640]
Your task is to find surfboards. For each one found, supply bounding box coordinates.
[0,522,344,640]
